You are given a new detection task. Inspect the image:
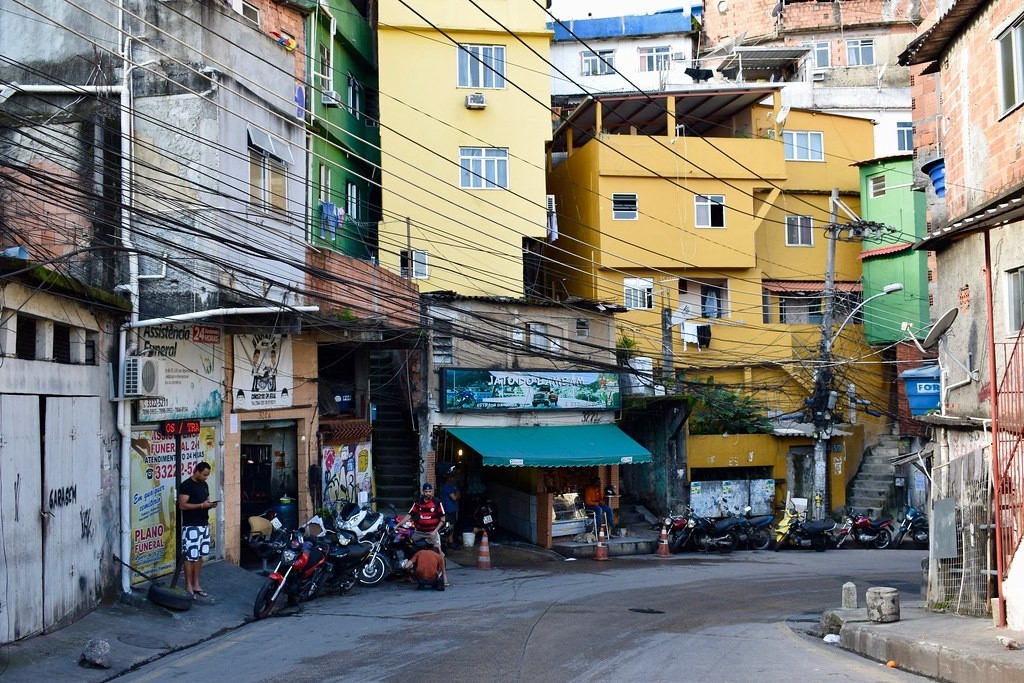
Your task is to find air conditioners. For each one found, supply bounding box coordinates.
[466,94,487,109]
[546,195,557,213]
[124,356,165,397]
[321,91,342,108]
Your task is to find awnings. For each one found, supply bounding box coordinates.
[442,423,652,467]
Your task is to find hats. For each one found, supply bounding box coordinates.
[423,482,432,491]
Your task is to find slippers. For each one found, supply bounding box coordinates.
[191,593,196,600]
[193,590,208,597]
[444,581,450,586]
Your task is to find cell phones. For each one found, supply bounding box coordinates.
[210,500,221,503]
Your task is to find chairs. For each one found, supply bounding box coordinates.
[247,516,284,575]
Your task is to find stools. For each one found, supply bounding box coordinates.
[582,499,610,542]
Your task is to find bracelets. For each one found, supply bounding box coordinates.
[202,503,204,509]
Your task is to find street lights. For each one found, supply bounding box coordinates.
[810,282,905,521]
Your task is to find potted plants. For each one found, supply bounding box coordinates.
[612,507,628,538]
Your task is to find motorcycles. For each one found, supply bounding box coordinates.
[309,526,378,603]
[359,517,407,587]
[774,497,838,552]
[390,513,456,558]
[834,506,895,550]
[476,504,498,540]
[244,516,334,620]
[658,503,775,554]
[334,484,384,541]
[894,503,930,549]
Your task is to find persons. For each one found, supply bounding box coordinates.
[392,482,449,586]
[442,474,461,548]
[405,543,443,591]
[585,475,618,531]
[179,462,218,599]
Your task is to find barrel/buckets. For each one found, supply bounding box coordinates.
[463,533,475,547]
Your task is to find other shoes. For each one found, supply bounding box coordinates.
[448,541,460,548]
[437,585,445,590]
[416,584,425,589]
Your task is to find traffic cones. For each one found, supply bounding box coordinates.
[653,525,675,558]
[592,527,613,561]
[477,531,491,569]
